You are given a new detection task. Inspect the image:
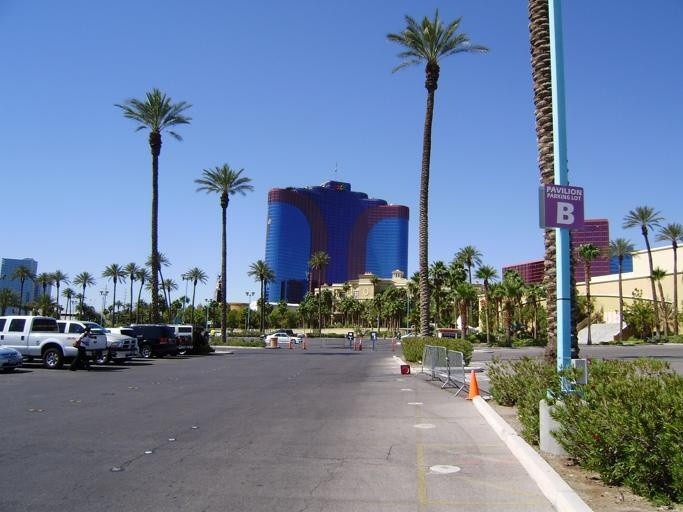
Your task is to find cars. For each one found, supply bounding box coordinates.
[0,314,210,371]
[263,330,306,345]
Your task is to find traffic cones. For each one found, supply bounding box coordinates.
[464,369,484,397]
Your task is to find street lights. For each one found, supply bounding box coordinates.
[99,288,109,327]
[244,289,255,332]
[181,272,193,322]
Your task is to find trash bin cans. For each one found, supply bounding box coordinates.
[271,338,278,347]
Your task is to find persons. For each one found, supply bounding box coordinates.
[77,328,92,371]
